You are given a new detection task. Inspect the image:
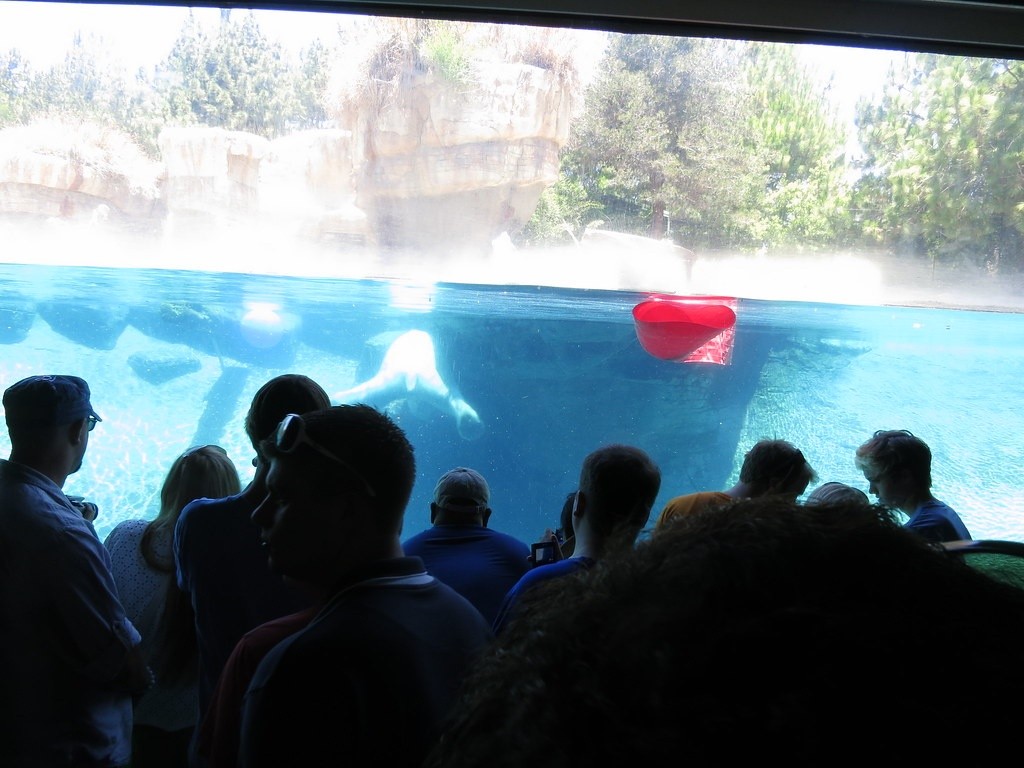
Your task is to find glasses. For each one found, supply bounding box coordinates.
[87,419,96,429]
[277,413,312,459]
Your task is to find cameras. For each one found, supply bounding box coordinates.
[66,496,99,521]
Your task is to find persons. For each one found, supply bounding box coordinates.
[174,376,333,767]
[241,405,498,768]
[421,499,1024,766]
[81,502,96,524]
[0,375,153,768]
[102,447,242,768]
[486,444,661,640]
[857,429,973,545]
[654,440,818,534]
[402,467,531,633]
[189,562,332,766]
[530,492,576,565]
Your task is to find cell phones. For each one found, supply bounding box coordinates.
[530,542,559,570]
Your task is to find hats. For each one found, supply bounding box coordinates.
[4,374,106,422]
